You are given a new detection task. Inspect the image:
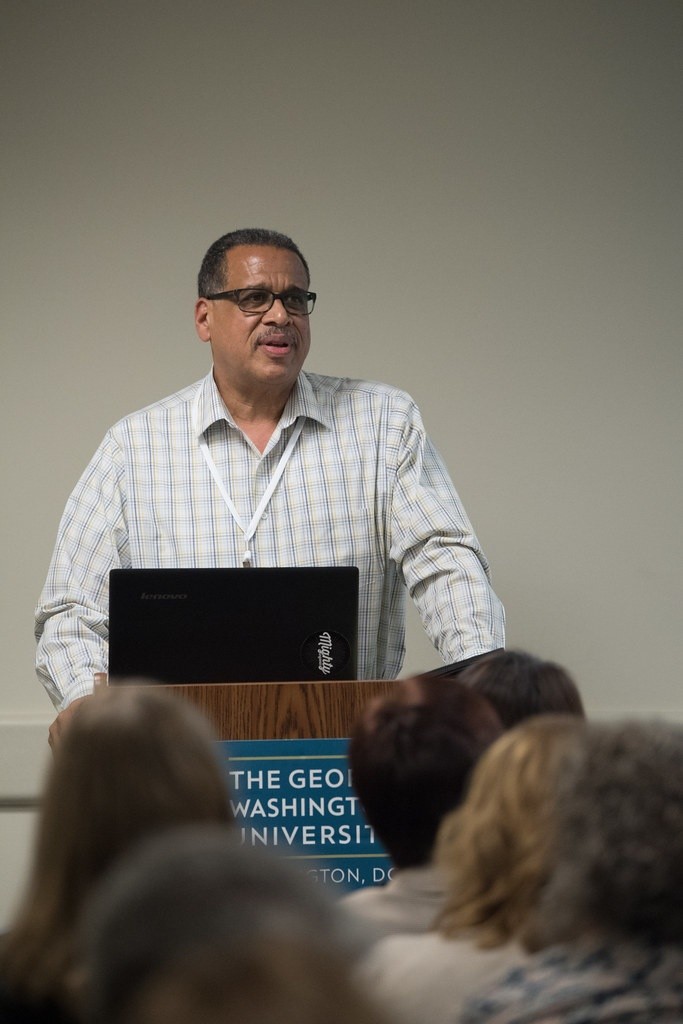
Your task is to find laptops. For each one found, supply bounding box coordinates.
[107,566,359,686]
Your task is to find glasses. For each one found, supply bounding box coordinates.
[205,285,317,317]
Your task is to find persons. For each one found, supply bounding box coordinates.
[0,649,682,1022]
[35,228,505,761]
